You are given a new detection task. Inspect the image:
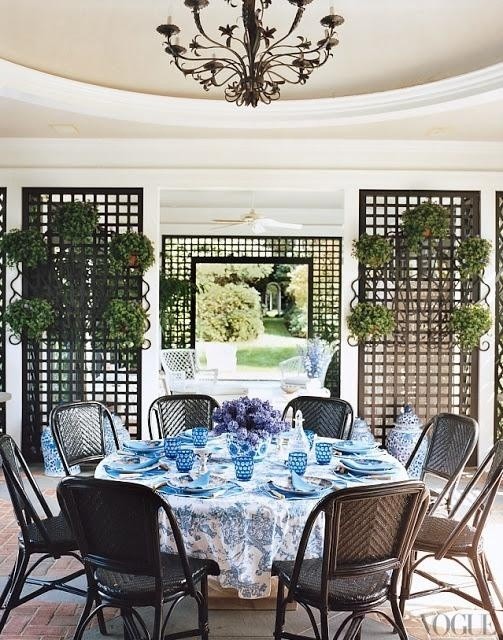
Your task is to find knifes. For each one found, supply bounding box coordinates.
[263,484,285,500]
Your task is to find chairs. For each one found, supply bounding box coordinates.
[274,479,430,639]
[1,433,111,636]
[49,400,121,479]
[55,478,219,640]
[394,439,503,639]
[281,396,354,439]
[280,356,325,390]
[160,349,218,395]
[147,394,221,439]
[403,409,479,516]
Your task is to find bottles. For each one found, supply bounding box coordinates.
[349,416,374,445]
[276,405,310,459]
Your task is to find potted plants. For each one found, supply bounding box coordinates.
[347,200,491,353]
[1,195,154,366]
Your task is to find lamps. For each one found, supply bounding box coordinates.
[156,0,344,108]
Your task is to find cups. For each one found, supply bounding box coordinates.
[164,427,254,482]
[288,430,333,478]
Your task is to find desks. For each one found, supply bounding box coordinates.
[194,379,331,418]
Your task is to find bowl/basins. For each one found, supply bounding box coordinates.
[280,384,300,394]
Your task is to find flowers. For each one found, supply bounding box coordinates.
[295,338,341,388]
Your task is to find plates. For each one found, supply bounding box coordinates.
[104,439,166,473]
[166,474,227,493]
[332,439,397,474]
[272,476,334,494]
